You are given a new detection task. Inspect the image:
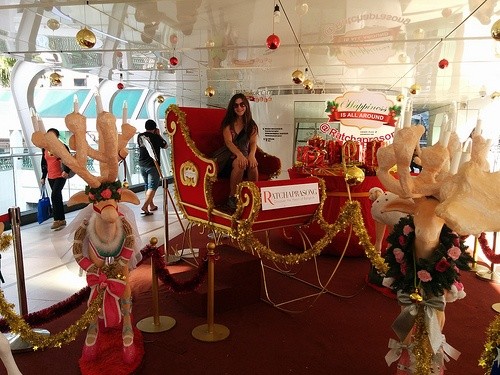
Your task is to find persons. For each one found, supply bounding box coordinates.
[40,128,72,230]
[212,93,259,209]
[137,119,168,216]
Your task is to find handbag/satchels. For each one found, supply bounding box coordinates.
[60,158,76,179]
[210,140,240,166]
[38,184,53,224]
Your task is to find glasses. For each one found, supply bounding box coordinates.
[232,102,245,108]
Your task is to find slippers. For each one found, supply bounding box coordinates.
[141,211,153,215]
[150,206,157,211]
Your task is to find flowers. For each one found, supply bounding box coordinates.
[86,180,123,202]
[381,213,477,296]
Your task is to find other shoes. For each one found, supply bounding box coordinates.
[227,196,236,211]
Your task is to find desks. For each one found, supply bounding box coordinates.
[287,168,424,258]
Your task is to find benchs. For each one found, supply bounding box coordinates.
[165,104,326,307]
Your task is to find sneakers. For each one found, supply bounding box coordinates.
[58,220,67,227]
[51,221,63,231]
